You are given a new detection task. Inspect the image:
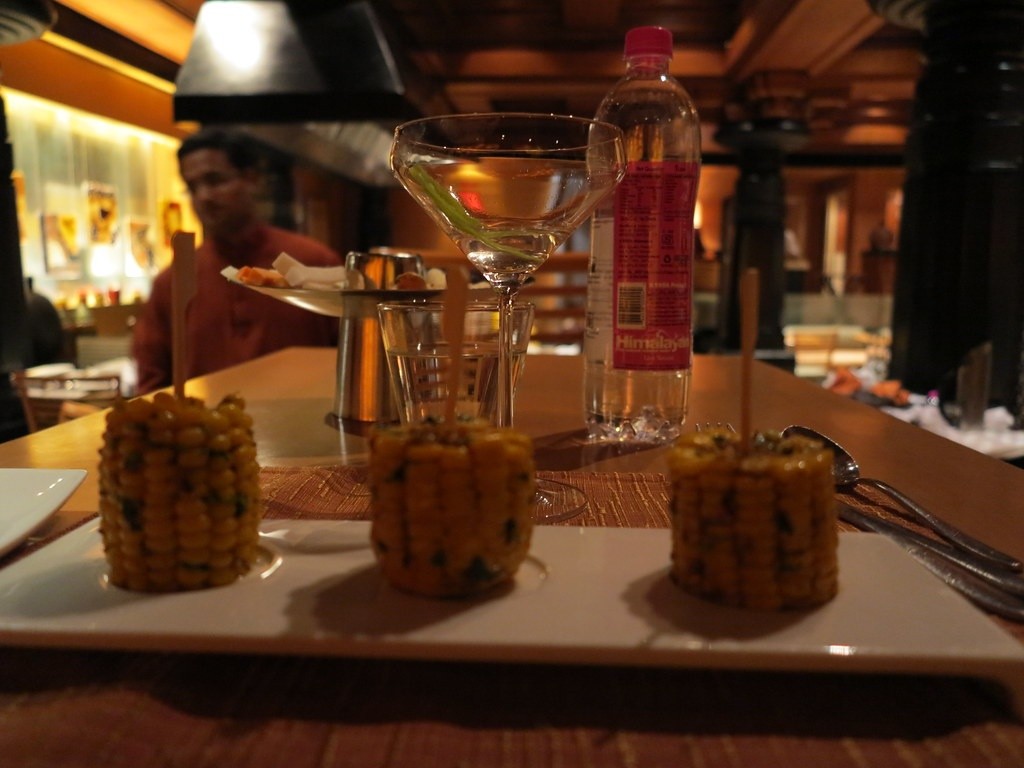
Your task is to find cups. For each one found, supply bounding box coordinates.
[378,301,534,426]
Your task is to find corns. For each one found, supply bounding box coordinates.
[668,429,840,610]
[98,390,260,593]
[369,414,536,596]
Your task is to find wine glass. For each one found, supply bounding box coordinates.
[389,113,629,523]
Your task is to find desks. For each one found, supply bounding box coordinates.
[0,348,1024,768]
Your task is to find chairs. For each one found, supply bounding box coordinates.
[11,371,122,433]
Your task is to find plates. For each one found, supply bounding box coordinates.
[219,266,444,317]
[0,514,1024,675]
[0,468,87,558]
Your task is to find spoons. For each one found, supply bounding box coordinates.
[780,425,1024,572]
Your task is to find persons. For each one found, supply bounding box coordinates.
[129,130,346,393]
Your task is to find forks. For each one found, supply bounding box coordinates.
[696,422,1024,621]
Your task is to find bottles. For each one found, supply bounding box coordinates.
[586,26,701,445]
[334,246,428,427]
